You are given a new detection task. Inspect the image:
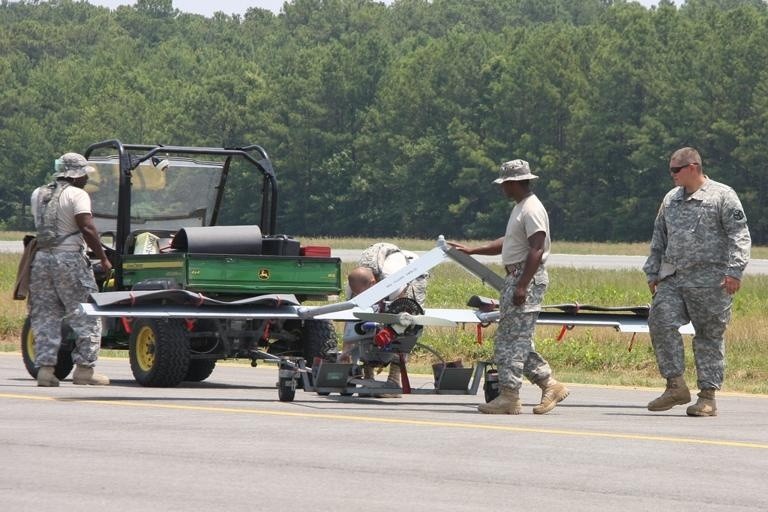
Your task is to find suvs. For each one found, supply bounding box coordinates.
[18,137,344,390]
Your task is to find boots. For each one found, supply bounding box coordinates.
[648,376,691,411]
[533,376,570,414]
[73,363,109,385]
[686,387,716,416]
[376,363,401,398]
[37,366,59,387]
[358,365,376,397]
[478,387,521,414]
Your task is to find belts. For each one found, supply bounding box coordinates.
[505,262,527,273]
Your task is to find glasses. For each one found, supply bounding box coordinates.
[671,163,699,173]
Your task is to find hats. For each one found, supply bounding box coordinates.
[491,160,540,183]
[52,153,95,178]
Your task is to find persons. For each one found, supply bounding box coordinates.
[342,241,431,400]
[446,158,569,414]
[641,148,752,417]
[30,151,111,387]
